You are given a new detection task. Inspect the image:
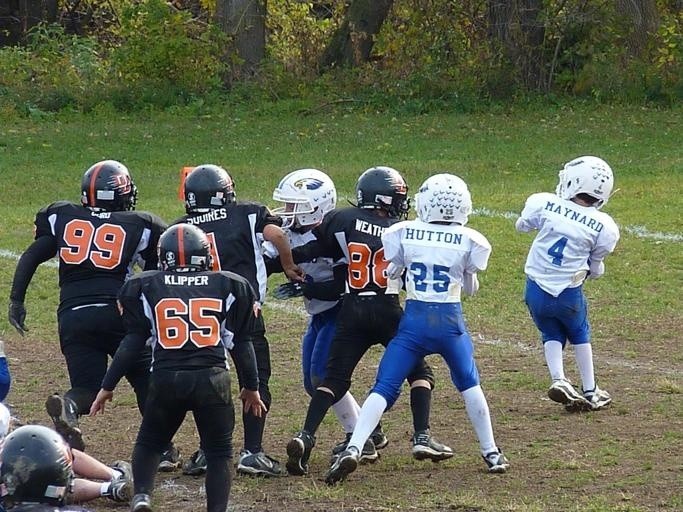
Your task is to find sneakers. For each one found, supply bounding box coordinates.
[285,432,316,476]
[325,422,390,481]
[413,428,455,462]
[483,449,509,472]
[184,448,208,474]
[548,380,612,411]
[157,444,182,472]
[45,396,86,452]
[101,460,149,512]
[238,450,282,478]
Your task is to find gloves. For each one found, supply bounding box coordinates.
[272,281,306,301]
[7,301,30,336]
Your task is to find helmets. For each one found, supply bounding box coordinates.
[557,156,614,210]
[355,166,410,221]
[157,222,213,275]
[416,172,473,225]
[81,160,138,212]
[272,169,337,231]
[3,425,75,507]
[185,164,235,215]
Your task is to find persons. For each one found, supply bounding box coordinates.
[254,169,388,465]
[89,222,266,511]
[515,156,619,413]
[0,341,134,505]
[285,167,454,475]
[8,159,185,471]
[166,164,305,477]
[325,173,510,488]
[0,425,91,512]
